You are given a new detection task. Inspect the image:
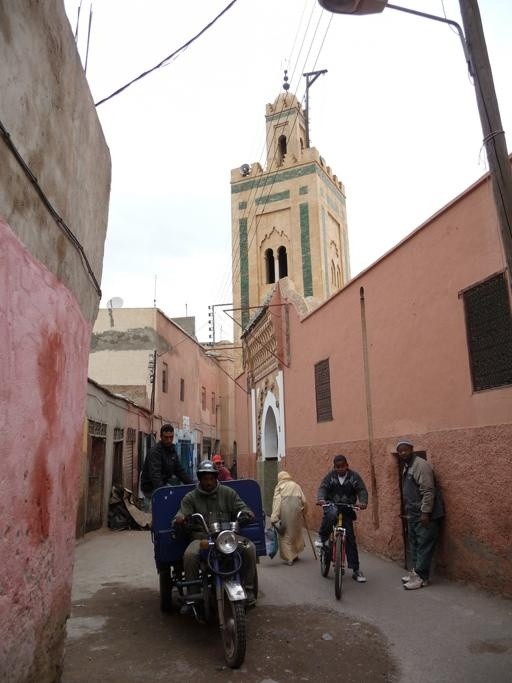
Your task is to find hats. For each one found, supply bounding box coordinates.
[396,439,414,452]
[196,455,225,472]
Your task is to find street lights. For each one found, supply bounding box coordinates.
[316,2,512,286]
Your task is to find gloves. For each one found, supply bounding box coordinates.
[237,512,250,523]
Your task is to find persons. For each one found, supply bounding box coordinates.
[169,460,257,616]
[268,470,307,565]
[141,424,194,497]
[315,454,369,581]
[213,454,231,478]
[395,439,443,590]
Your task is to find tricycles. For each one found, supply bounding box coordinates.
[151,478,267,670]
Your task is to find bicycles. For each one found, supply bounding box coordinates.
[315,500,362,601]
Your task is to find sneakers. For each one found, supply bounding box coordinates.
[401,568,428,590]
[352,570,367,581]
[314,536,323,547]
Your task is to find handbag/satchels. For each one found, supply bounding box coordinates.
[264,528,278,560]
